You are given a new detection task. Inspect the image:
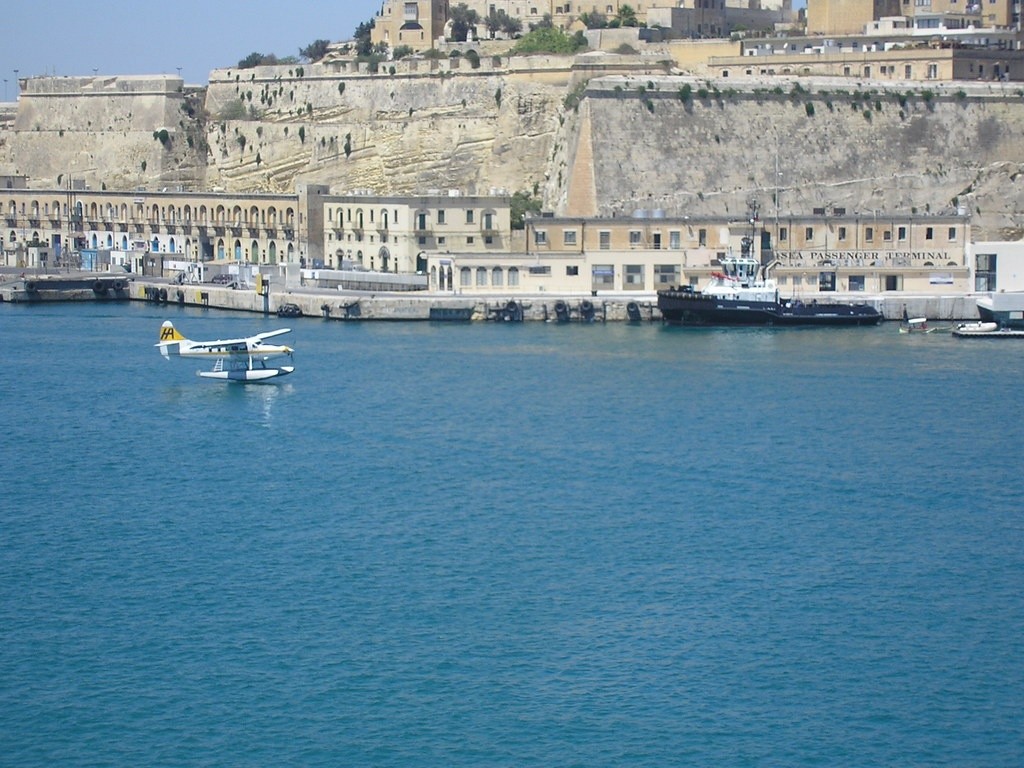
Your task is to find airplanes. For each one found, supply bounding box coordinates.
[154,321,295,380]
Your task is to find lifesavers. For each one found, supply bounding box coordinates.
[626,302,638,313]
[25,281,37,293]
[159,288,167,299]
[277,304,300,317]
[151,288,159,298]
[507,301,517,313]
[93,281,106,293]
[113,279,123,291]
[581,301,593,312]
[555,302,566,313]
[177,290,184,300]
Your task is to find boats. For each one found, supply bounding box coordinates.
[898,318,954,335]
[657,198,881,325]
[956,321,997,332]
